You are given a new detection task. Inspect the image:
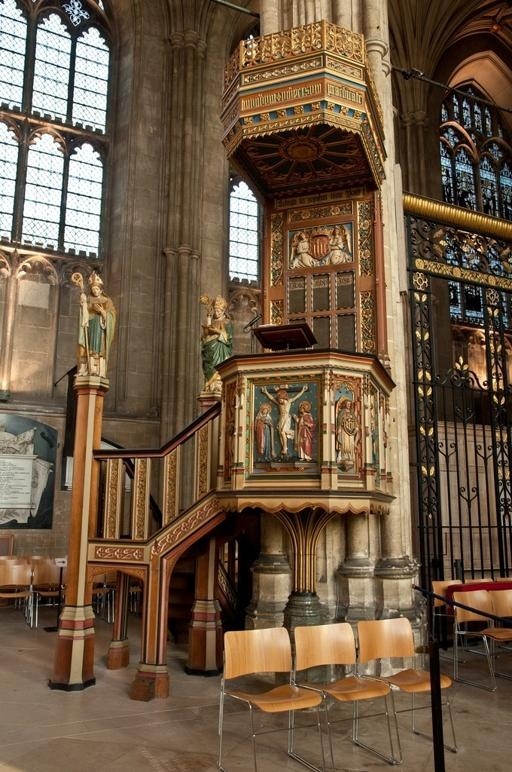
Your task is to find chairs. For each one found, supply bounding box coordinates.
[290,622,398,771]
[429,578,512,694]
[356,616,458,764]
[218,626,328,771]
[0,555,145,630]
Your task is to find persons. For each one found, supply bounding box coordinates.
[292,231,316,269]
[295,402,314,461]
[254,404,274,463]
[72,271,116,377]
[261,384,309,455]
[199,294,232,391]
[321,225,353,264]
[337,399,360,465]
